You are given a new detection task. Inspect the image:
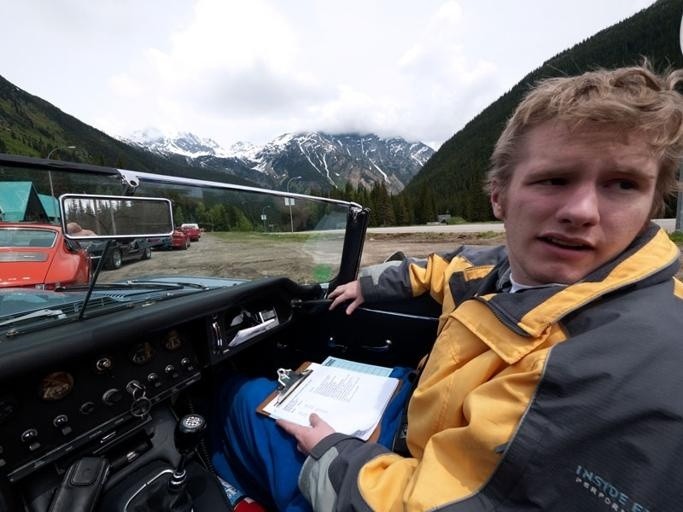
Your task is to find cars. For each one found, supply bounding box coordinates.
[84,239,152,271]
[152,223,201,250]
[0,223,92,289]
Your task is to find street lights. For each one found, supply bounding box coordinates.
[263,206,270,232]
[287,176,302,232]
[47,145,76,224]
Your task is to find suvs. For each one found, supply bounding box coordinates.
[0,153,442,511]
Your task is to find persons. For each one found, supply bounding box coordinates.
[208,55,683,512]
